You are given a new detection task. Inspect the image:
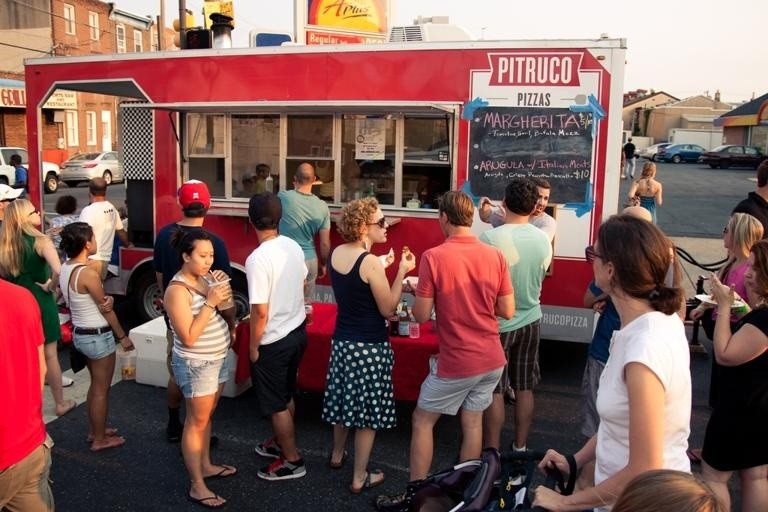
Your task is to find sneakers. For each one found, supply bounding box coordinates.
[255,436,282,459]
[256,457,307,481]
[376,493,406,511]
[44,375,74,387]
[167,423,183,444]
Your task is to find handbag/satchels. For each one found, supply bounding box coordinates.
[622,193,641,208]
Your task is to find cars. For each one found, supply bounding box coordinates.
[0,146,61,194]
[640,140,765,171]
[61,151,124,186]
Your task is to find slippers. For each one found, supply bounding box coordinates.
[185,492,225,508]
[330,448,348,468]
[86,428,125,450]
[202,464,239,478]
[349,467,384,492]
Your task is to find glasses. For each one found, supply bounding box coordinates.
[722,225,728,235]
[0,198,16,202]
[366,216,386,228]
[29,209,38,215]
[584,245,605,263]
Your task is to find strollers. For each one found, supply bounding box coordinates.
[375,446,578,511]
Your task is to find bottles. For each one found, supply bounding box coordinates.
[398,297,410,336]
[265,172,274,195]
[390,313,398,336]
[368,183,376,197]
[409,310,419,338]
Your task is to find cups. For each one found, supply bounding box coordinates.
[207,279,234,311]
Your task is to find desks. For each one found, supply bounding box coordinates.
[235,301,441,419]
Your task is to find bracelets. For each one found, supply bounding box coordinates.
[203,302,216,312]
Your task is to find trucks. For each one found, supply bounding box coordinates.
[23,38,628,345]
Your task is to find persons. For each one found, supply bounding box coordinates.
[153,178,237,449]
[320,197,418,495]
[532,206,766,512]
[628,162,663,224]
[0,155,77,511]
[731,160,768,249]
[298,122,334,199]
[162,227,237,509]
[410,177,558,478]
[245,190,310,482]
[49,177,134,449]
[276,161,332,297]
[623,138,636,179]
[233,164,274,198]
[403,118,450,210]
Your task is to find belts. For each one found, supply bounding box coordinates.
[72,325,112,335]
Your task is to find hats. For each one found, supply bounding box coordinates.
[177,179,210,214]
[0,183,24,202]
